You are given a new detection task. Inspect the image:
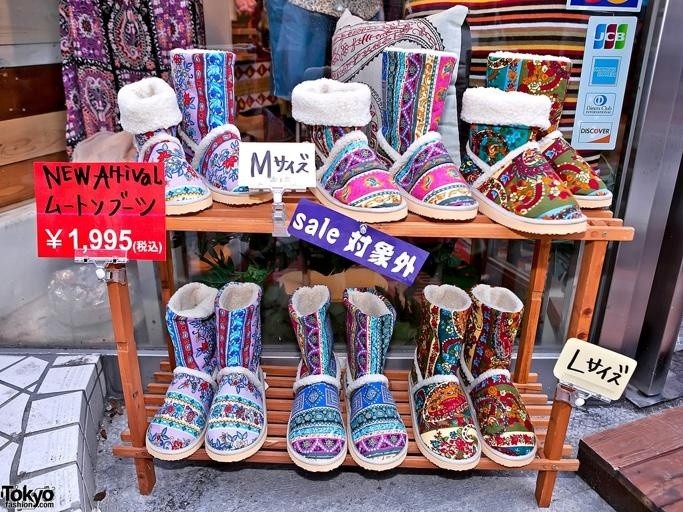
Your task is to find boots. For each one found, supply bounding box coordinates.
[457,85,590,236]
[406,283,482,474]
[340,285,411,473]
[168,46,275,207]
[485,50,614,210]
[455,282,540,469]
[374,46,482,223]
[144,280,220,463]
[285,283,349,475]
[203,280,266,464]
[289,76,410,224]
[114,75,214,215]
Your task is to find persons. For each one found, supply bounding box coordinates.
[263,0,384,101]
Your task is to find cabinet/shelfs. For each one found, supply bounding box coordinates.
[99,186,636,509]
